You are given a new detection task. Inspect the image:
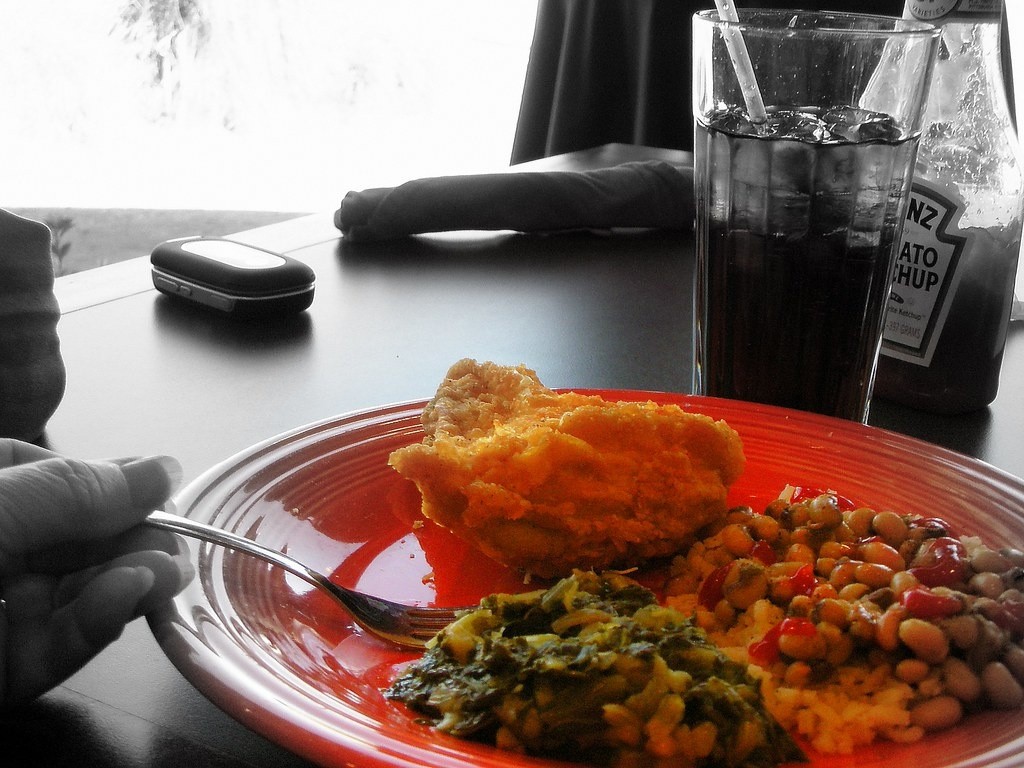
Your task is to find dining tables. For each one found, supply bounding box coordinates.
[0,202,1024,768]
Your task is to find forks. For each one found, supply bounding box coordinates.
[141,509,497,654]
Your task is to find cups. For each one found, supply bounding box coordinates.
[692,6,943,427]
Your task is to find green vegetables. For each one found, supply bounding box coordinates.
[381,563,813,768]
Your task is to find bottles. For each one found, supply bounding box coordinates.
[847,0,1024,415]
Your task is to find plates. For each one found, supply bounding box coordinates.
[141,388,1024,768]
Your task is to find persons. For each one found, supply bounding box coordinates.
[0,437,191,718]
